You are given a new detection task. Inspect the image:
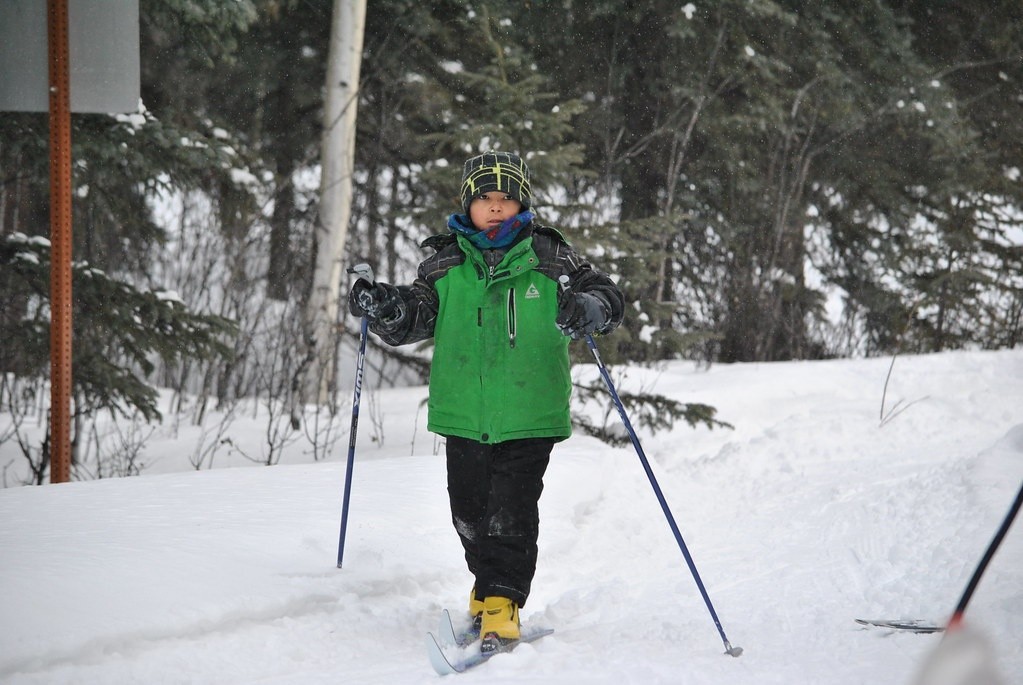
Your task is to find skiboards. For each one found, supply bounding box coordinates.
[423,608,555,676]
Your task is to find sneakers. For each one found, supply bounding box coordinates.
[469,585,485,630]
[479,596,521,650]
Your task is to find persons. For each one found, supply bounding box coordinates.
[348,150,626,660]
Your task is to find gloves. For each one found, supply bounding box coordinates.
[348,277,399,323]
[554,291,608,340]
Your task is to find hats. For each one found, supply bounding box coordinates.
[461,151,532,218]
